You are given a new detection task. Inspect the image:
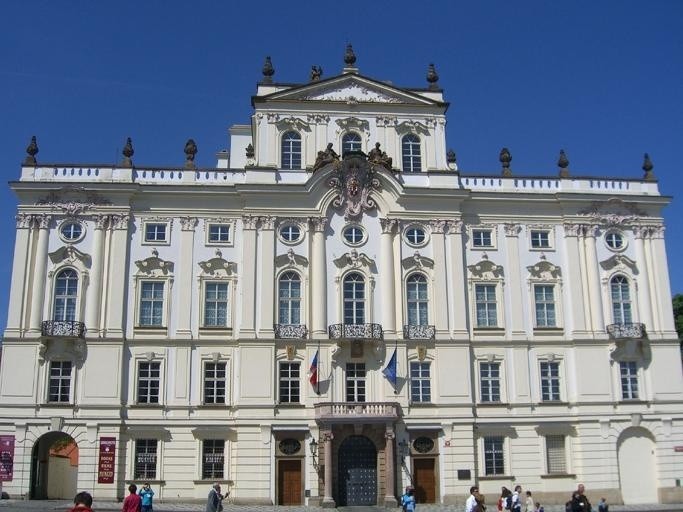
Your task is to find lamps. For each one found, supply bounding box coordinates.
[398,438,408,456]
[308,436,318,456]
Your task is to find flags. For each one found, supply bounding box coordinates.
[380,346,396,384]
[307,350,319,392]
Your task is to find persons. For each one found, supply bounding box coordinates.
[397,486,418,511]
[216,487,230,512]
[465,486,480,512]
[120,483,142,512]
[203,481,221,512]
[497,486,544,511]
[138,482,154,511]
[62,491,94,511]
[597,496,609,512]
[571,490,584,512]
[474,493,487,511]
[578,484,592,511]
